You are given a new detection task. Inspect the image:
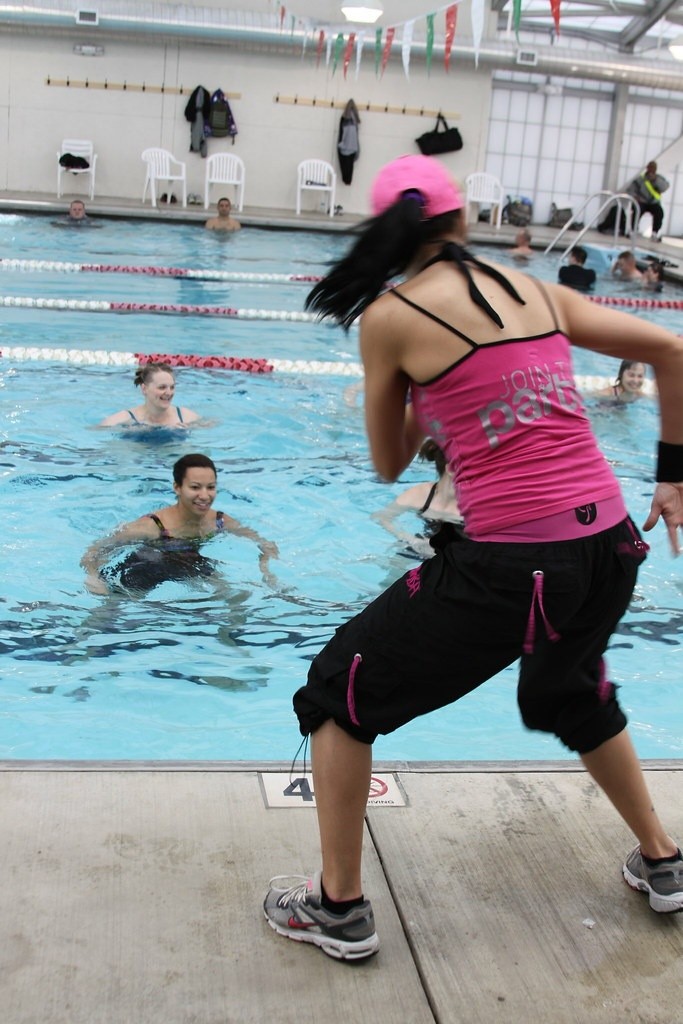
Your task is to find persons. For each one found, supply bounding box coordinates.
[596,360,646,397]
[559,247,596,289]
[206,198,240,229]
[102,363,196,428]
[626,161,669,241]
[612,252,641,279]
[512,231,532,253]
[132,456,234,531]
[642,263,664,282]
[70,200,85,219]
[263,154,683,959]
[398,439,460,516]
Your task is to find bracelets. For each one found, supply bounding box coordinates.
[655,441,683,483]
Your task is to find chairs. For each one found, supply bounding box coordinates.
[463,171,506,229]
[55,139,99,202]
[203,152,245,213]
[296,159,337,218]
[140,147,187,208]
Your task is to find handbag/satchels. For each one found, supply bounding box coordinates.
[598,206,625,236]
[549,203,574,229]
[338,110,358,155]
[502,196,531,226]
[416,116,463,156]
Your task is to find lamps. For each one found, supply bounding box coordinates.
[340,0,384,24]
[668,34,683,60]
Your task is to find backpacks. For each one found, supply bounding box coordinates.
[206,89,230,138]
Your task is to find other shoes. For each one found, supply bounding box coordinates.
[188,194,203,204]
[329,205,345,216]
[651,234,661,242]
[161,194,177,203]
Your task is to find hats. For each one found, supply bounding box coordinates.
[370,154,465,218]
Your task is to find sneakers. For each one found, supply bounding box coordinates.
[262,871,380,959]
[623,836,683,913]
